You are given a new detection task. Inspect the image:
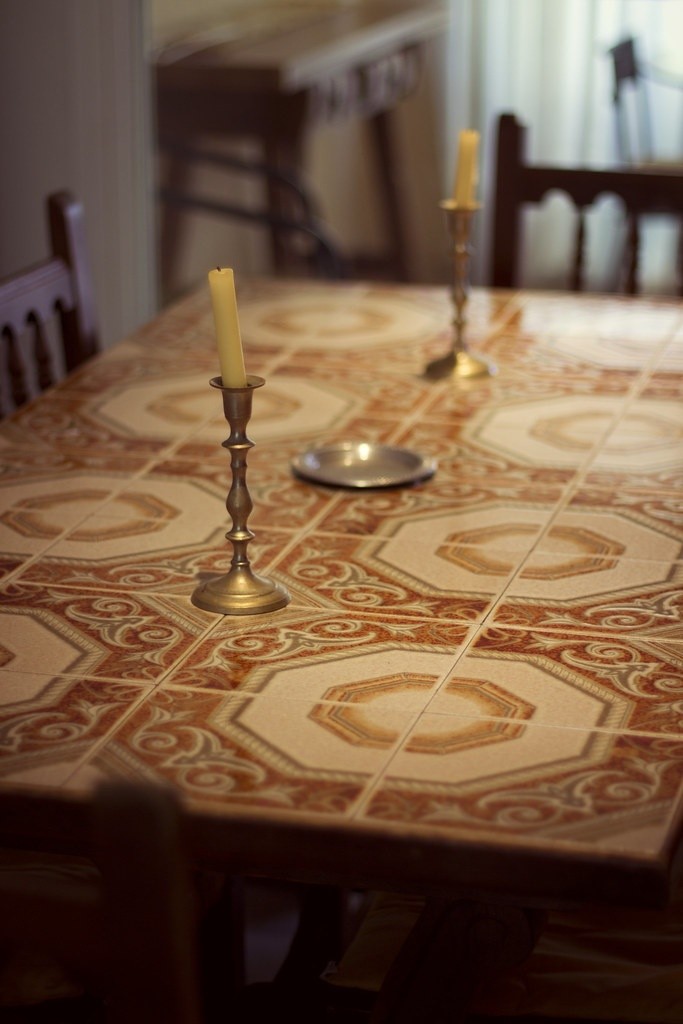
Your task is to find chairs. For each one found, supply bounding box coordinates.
[489,112,682,296]
[1,189,104,420]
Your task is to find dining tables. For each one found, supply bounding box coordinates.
[1,272,683,1024]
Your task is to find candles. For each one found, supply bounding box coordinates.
[455,130,479,208]
[208,265,247,389]
